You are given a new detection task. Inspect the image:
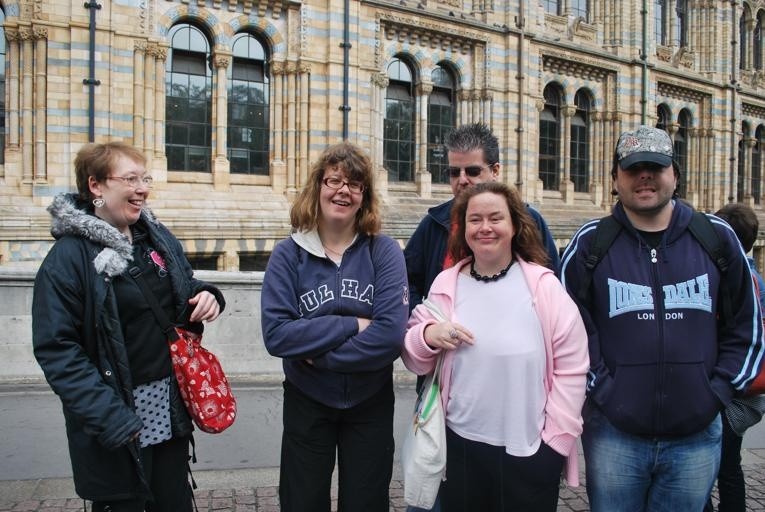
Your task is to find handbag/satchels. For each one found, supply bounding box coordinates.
[404,381,447,510]
[169,327,236,433]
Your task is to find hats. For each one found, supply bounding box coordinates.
[620,126,676,170]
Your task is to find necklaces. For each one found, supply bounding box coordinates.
[469,256,511,283]
[321,242,345,257]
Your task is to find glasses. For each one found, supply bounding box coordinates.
[106,176,155,188]
[322,177,364,194]
[443,164,494,178]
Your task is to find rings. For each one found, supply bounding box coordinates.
[451,328,458,339]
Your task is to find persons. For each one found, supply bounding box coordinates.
[404,124,561,511]
[260,139,410,511]
[33,140,225,512]
[400,176,589,512]
[708,202,763,511]
[559,124,763,511]
